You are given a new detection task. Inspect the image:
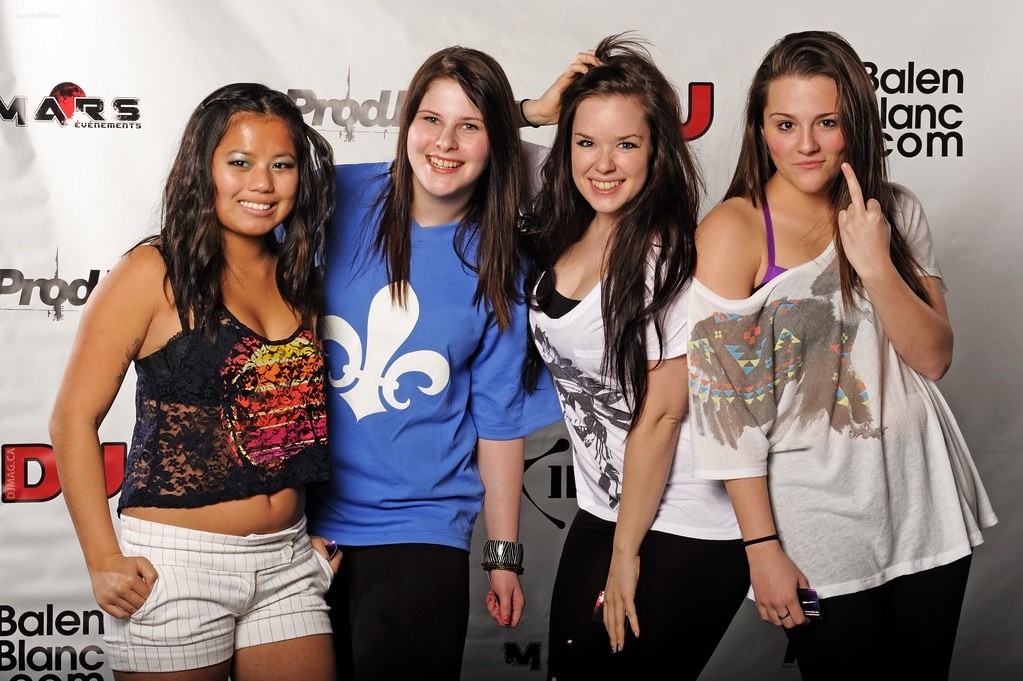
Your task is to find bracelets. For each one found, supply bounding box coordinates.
[743,535,778,546]
[481,562,524,575]
[520,99,541,128]
[482,540,524,564]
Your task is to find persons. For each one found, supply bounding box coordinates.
[688,31,997,681]
[516,32,750,680]
[47,82,343,680]
[274,45,562,681]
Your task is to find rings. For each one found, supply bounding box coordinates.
[779,613,789,620]
